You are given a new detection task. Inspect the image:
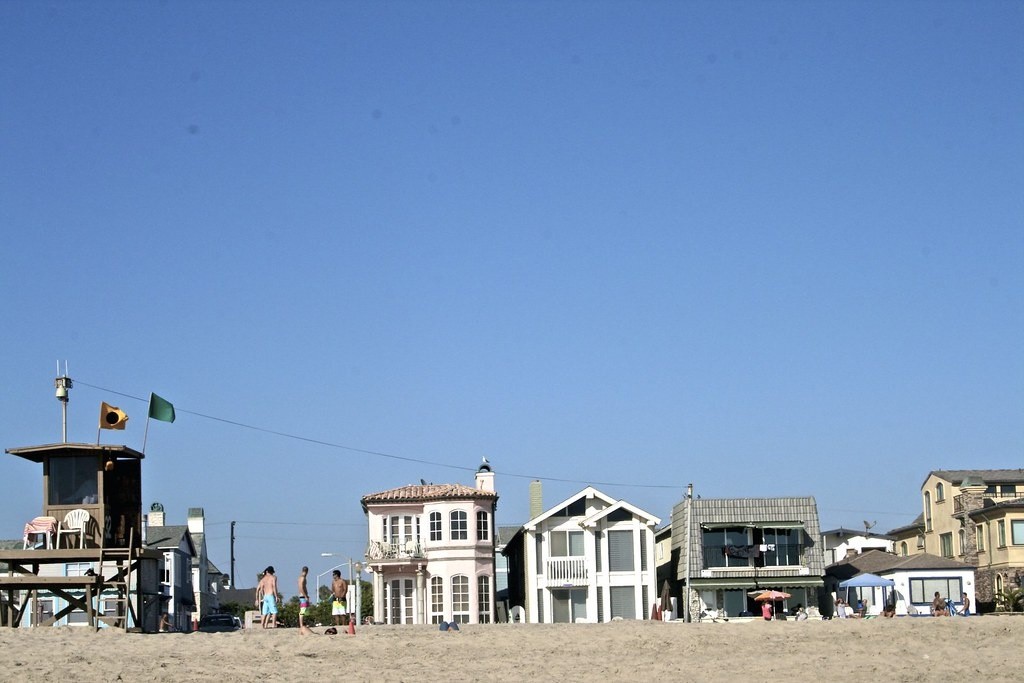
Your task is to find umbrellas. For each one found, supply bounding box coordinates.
[839,572,895,587]
[661,579,673,622]
[747,589,771,598]
[754,590,793,620]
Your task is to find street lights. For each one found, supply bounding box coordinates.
[321,553,353,618]
[316,561,368,602]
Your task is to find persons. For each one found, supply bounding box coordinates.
[931,591,950,617]
[855,599,867,618]
[833,598,850,618]
[298,626,337,635]
[297,566,309,628]
[331,570,348,626]
[956,592,970,617]
[879,605,896,619]
[159,612,174,632]
[795,607,808,621]
[254,566,280,629]
[761,599,772,621]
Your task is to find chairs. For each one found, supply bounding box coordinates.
[57,508,91,550]
[23,516,56,549]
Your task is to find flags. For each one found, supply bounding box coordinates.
[148,392,175,423]
[100,401,129,430]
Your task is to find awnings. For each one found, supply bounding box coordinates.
[753,521,805,528]
[690,578,756,590]
[755,577,824,587]
[701,522,755,528]
